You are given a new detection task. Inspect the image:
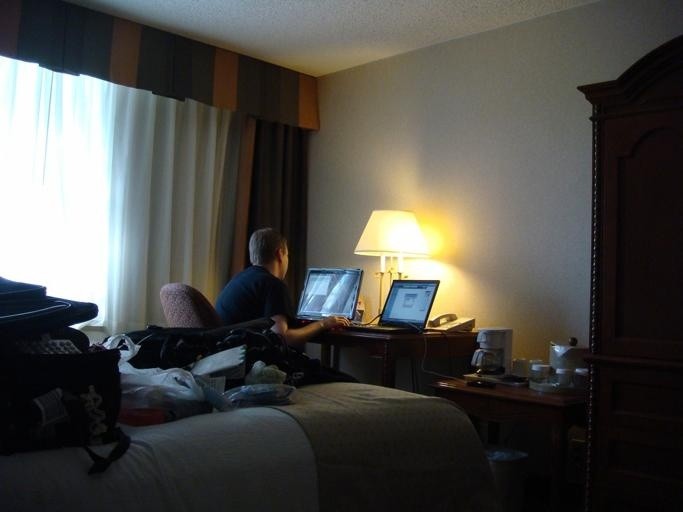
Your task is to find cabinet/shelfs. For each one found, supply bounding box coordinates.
[575,36,681,511]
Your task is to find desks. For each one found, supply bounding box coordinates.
[423,378,587,510]
[287,322,478,393]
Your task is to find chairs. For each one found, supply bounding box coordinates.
[159,282,226,331]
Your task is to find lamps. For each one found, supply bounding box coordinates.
[352,209,430,317]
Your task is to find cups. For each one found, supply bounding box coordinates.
[531,364,550,384]
[554,368,588,386]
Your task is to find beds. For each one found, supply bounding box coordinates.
[0,382,495,511]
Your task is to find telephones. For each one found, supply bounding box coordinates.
[428,313,475,333]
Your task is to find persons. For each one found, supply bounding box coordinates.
[215,226,360,384]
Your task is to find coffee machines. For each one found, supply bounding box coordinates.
[463,327,513,386]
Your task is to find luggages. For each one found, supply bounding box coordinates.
[0,277,121,456]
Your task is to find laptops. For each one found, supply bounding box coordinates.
[296,267,361,322]
[345,279,440,330]
